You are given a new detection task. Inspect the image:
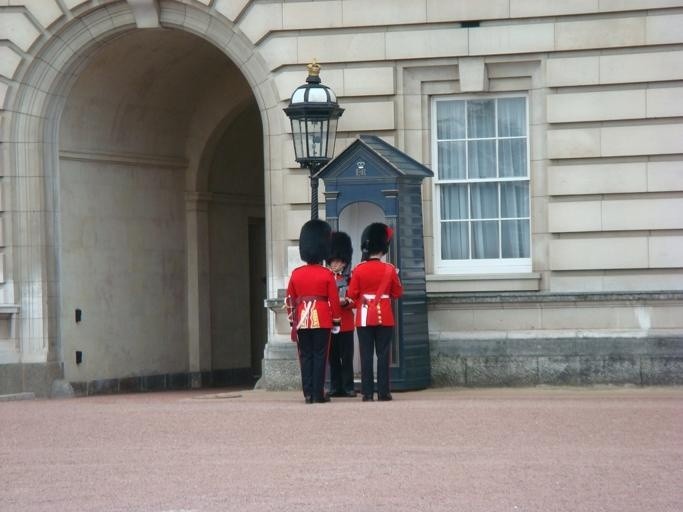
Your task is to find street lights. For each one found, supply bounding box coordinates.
[282,54,345,221]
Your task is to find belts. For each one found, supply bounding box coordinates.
[364,294,389,299]
[296,296,328,303]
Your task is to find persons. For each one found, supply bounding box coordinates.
[344,244,403,403]
[283,259,342,405]
[324,257,359,399]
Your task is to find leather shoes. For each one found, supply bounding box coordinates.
[305,390,392,403]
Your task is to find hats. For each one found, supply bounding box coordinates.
[326,231,352,264]
[299,220,331,264]
[361,222,390,255]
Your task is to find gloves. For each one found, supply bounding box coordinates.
[331,326,340,334]
